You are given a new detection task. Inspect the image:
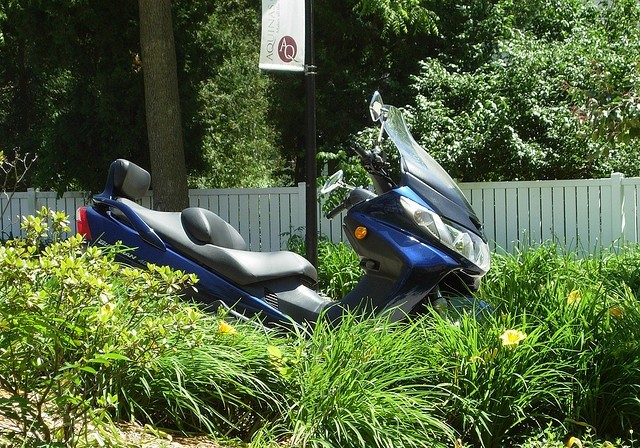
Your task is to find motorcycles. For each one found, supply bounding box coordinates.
[75,90,491,338]
